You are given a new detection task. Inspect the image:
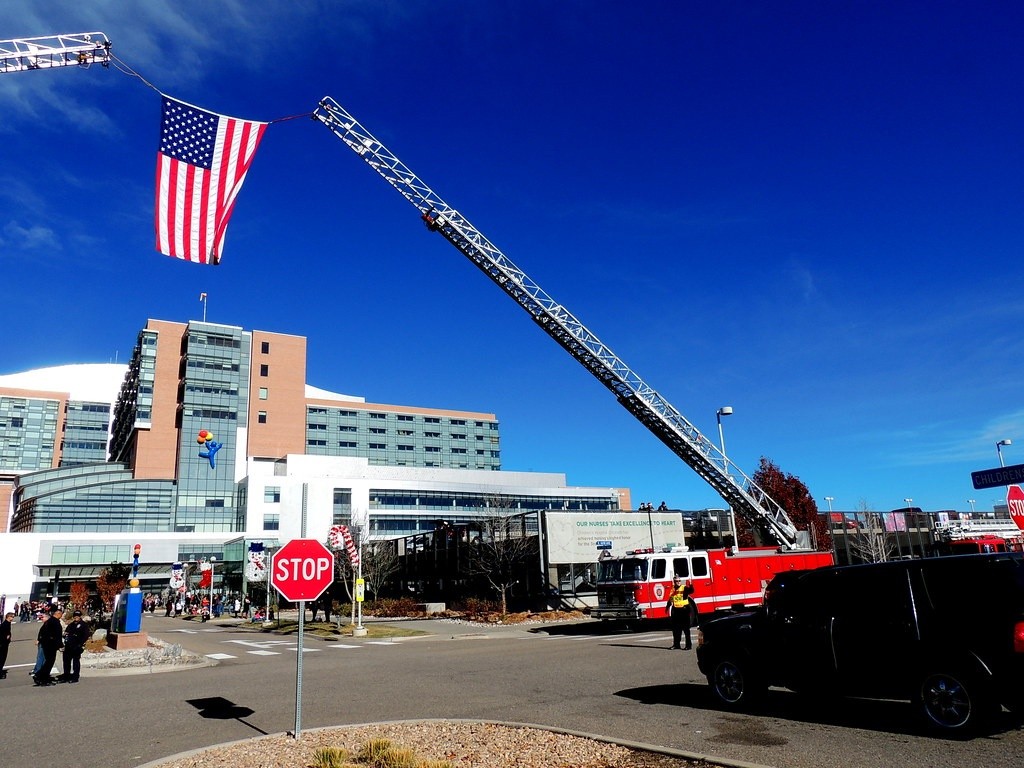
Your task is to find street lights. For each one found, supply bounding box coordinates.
[995,439,1011,492]
[967,500,976,512]
[824,496,833,512]
[716,405,739,549]
[904,498,912,508]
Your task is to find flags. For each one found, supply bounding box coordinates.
[156,93,269,265]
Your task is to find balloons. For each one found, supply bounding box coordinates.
[199,430,207,438]
[197,436,206,444]
[206,432,213,440]
[198,440,223,469]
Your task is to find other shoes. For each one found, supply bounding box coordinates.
[29,672,34,675]
[684,646,692,650]
[69,678,78,683]
[0,673,6,679]
[670,645,680,650]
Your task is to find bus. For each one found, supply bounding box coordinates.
[949,534,1008,555]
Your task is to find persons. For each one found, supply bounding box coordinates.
[28,611,91,687]
[165,592,225,623]
[639,501,668,511]
[0,613,16,679]
[143,594,163,615]
[13,600,70,623]
[226,592,275,623]
[665,575,695,650]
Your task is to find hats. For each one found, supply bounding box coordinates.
[73,610,82,617]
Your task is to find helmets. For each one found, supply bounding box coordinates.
[673,577,681,580]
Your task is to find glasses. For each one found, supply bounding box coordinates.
[675,580,681,582]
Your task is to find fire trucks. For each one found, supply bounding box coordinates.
[306,94,837,627]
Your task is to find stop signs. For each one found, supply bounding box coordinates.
[269,538,334,601]
[1006,484,1024,531]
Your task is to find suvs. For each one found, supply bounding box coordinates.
[693,549,1023,738]
